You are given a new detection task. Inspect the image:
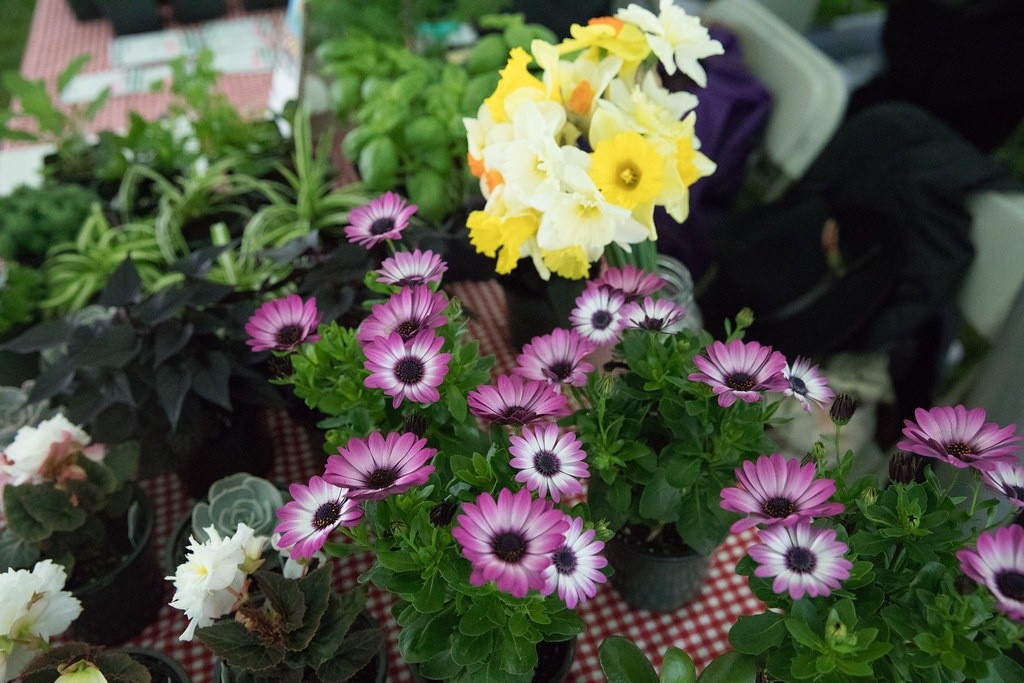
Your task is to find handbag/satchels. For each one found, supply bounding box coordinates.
[697,101,982,453]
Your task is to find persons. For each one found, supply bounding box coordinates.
[515,0,1024,454]
